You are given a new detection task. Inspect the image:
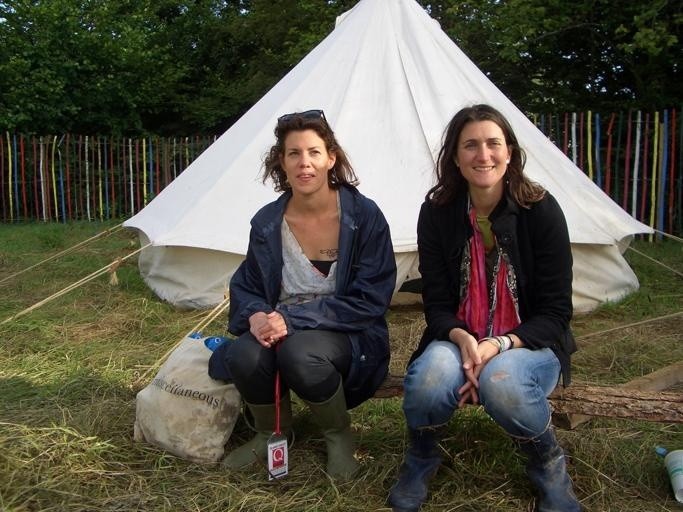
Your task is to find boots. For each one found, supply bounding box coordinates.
[222,398,293,472]
[307,376,358,480]
[499,415,580,512]
[387,426,449,512]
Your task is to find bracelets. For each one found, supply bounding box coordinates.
[478,335,514,353]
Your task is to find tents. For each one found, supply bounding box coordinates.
[122,0,655,318]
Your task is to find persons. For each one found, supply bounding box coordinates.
[209,110,398,479]
[389,104,581,511]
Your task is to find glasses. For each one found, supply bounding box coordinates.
[278,110,327,123]
